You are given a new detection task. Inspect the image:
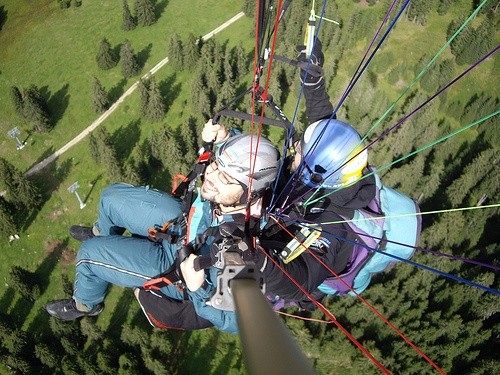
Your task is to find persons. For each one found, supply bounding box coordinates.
[129,35,384,333]
[42,117,282,320]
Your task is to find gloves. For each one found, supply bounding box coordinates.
[296,35,324,84]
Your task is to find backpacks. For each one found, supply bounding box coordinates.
[312,167,422,298]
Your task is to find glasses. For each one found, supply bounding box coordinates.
[210,156,241,185]
[294,137,303,159]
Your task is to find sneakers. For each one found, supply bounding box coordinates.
[69,225,95,241]
[45,298,105,321]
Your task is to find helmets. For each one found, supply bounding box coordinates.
[298,119,369,189]
[216,133,281,202]
